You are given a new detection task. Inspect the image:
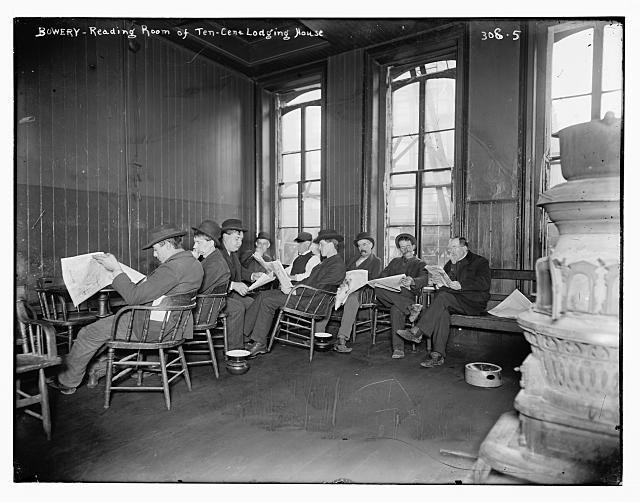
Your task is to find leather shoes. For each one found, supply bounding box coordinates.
[393,349,405,359]
[46,373,77,394]
[247,342,268,357]
[422,351,444,368]
[408,303,423,322]
[334,338,354,353]
[397,326,425,343]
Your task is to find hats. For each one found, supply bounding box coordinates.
[354,232,375,247]
[256,232,271,242]
[189,220,221,246]
[395,232,417,248]
[314,229,343,245]
[295,232,312,242]
[141,224,184,251]
[222,218,248,232]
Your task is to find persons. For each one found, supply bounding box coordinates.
[217,219,255,351]
[396,235,491,368]
[375,233,428,358]
[304,233,382,353]
[239,231,273,299]
[45,222,204,395]
[243,229,347,358]
[130,220,232,379]
[284,231,321,281]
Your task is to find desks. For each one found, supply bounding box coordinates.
[86,289,116,388]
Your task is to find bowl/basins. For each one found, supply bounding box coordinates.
[465,362,502,388]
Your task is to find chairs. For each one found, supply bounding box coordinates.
[15,302,61,442]
[161,284,228,387]
[371,291,423,352]
[34,279,99,365]
[212,315,229,362]
[268,281,340,362]
[329,286,375,343]
[104,288,196,412]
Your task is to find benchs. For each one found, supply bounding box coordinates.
[423,269,537,353]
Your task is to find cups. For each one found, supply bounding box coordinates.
[315,332,332,351]
[225,350,251,375]
